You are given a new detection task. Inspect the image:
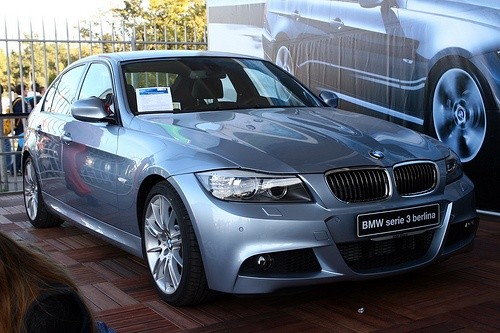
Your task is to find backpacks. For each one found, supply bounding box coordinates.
[4,98,22,135]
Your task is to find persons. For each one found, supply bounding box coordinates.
[0,83,46,176]
[0,232,98,333]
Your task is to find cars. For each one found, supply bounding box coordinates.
[20,50,479,306]
[263,0,500,173]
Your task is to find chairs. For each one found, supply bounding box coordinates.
[191,78,238,111]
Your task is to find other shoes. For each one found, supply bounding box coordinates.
[12,170,22,176]
